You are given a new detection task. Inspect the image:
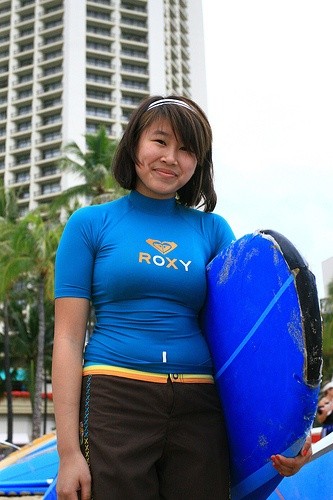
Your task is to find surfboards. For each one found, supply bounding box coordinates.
[200,230,324,500]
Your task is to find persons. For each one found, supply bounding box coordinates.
[315,381,333,440]
[51,96,312,499]
